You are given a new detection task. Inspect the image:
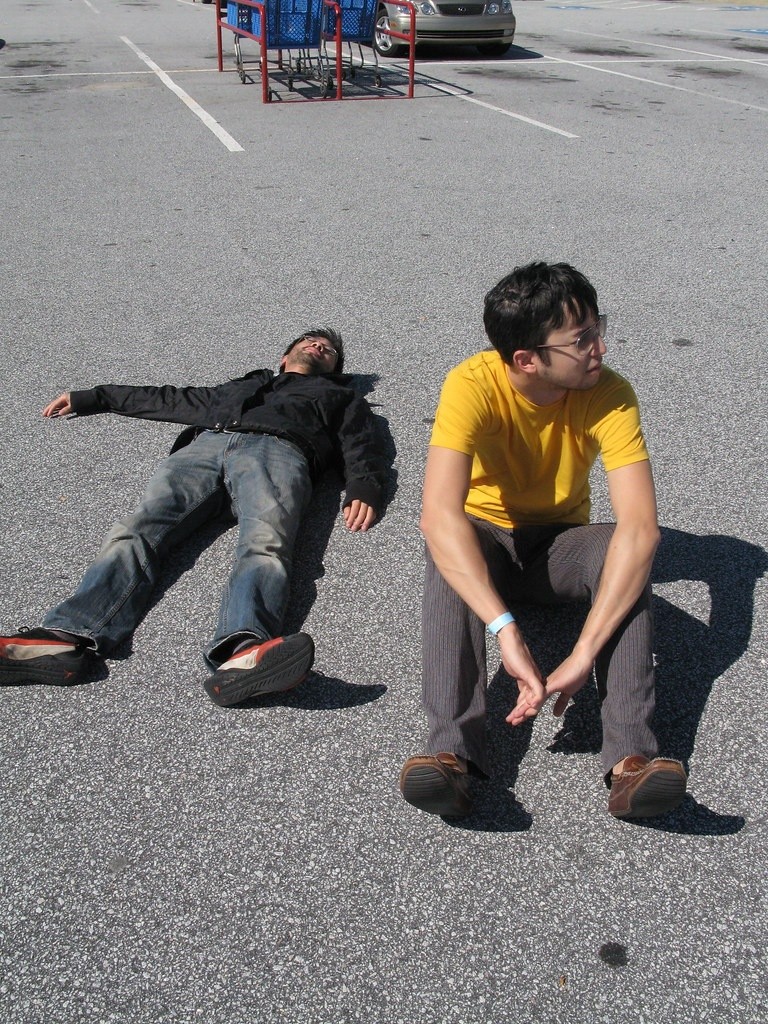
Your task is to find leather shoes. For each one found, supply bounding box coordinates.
[607,756,687,819]
[400,752,473,819]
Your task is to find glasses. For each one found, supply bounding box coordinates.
[509,314,607,363]
[294,335,338,363]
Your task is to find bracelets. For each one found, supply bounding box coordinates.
[489,612,517,637]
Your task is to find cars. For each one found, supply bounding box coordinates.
[362,0,517,58]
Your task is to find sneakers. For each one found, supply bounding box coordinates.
[204,633,315,707]
[0,626,91,686]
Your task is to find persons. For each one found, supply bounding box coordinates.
[400,261,688,822]
[0,327,389,708]
